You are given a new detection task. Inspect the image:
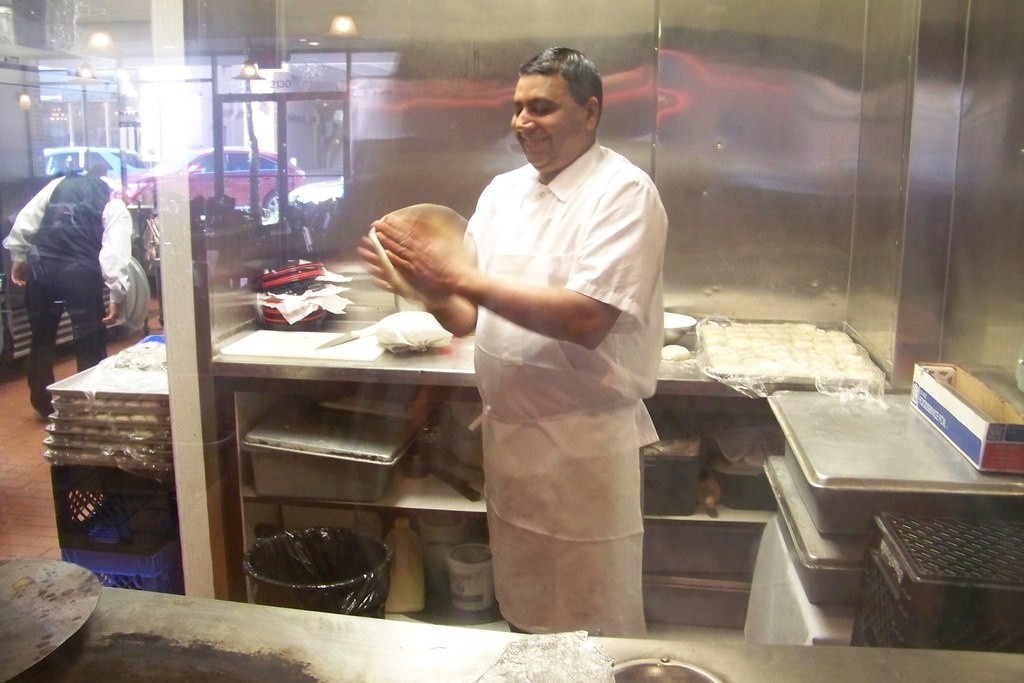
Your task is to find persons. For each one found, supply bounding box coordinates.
[359,46,669,640]
[2,174,133,416]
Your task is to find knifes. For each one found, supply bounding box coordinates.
[426,466,481,502]
[318,323,377,348]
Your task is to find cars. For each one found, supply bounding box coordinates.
[285,176,345,206]
[111,148,306,217]
[44,147,155,178]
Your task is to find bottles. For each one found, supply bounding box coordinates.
[399,449,429,479]
[383,516,426,613]
[416,425,444,468]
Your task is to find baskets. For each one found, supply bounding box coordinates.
[258,262,328,331]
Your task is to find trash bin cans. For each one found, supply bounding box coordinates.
[242,526,392,618]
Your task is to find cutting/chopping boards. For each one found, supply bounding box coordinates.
[220,327,387,361]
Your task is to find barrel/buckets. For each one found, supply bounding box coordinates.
[416,512,465,585]
[444,543,495,611]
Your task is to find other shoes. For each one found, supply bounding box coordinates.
[39,406,54,417]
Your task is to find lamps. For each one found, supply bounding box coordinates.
[230,39,267,81]
[19,93,31,109]
[321,15,363,39]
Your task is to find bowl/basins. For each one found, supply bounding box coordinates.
[664,311,697,346]
[444,397,482,468]
[261,261,329,331]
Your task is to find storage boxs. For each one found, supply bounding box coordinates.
[60,539,185,596]
[910,362,1024,475]
[850,512,1024,654]
[50,463,179,557]
